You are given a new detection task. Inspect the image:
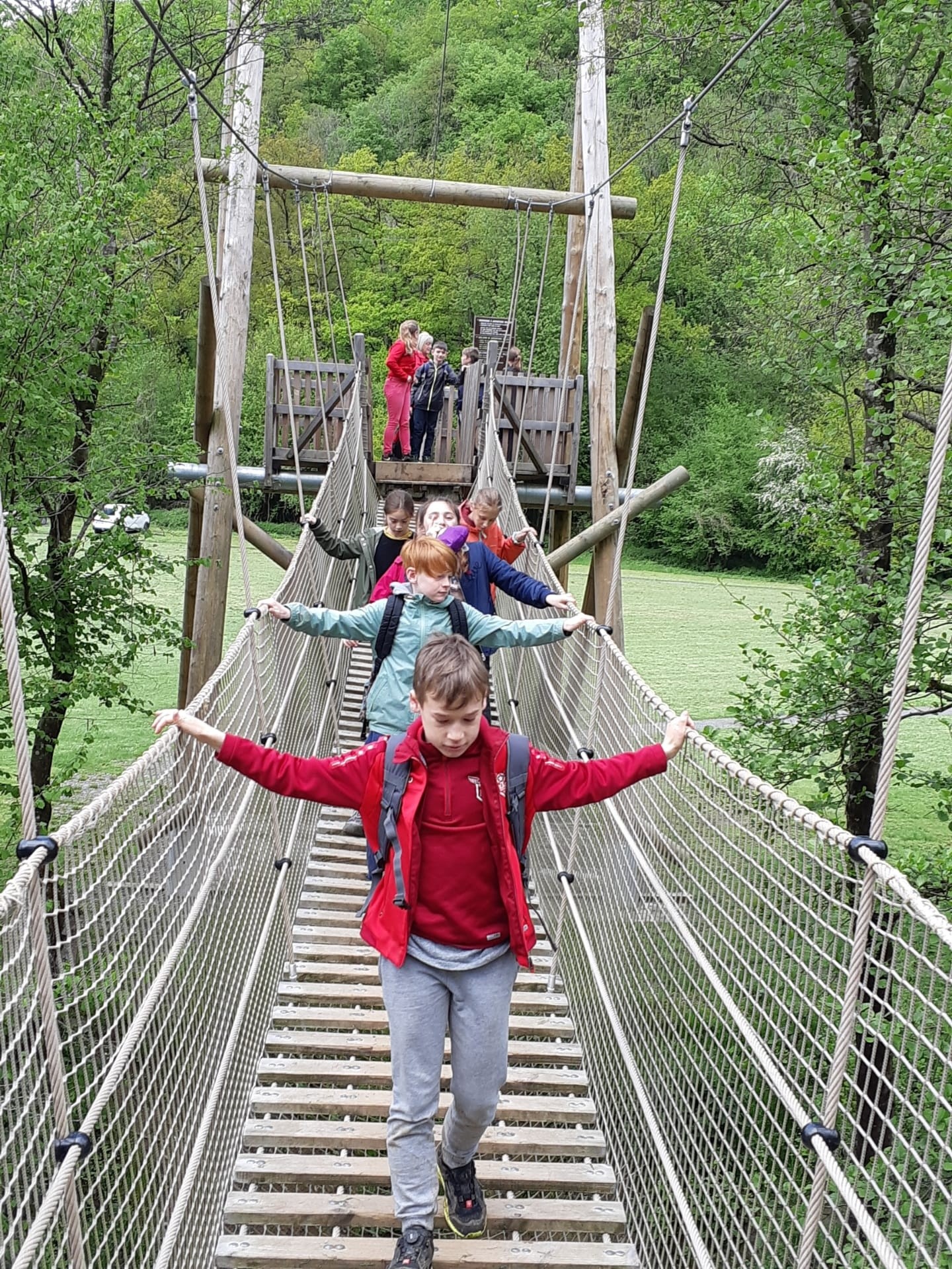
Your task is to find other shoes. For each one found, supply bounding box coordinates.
[342,810,364,836]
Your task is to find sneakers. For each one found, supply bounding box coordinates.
[381,455,398,461]
[410,454,419,461]
[390,1226,434,1269]
[402,454,411,461]
[434,1141,488,1238]
[423,455,433,462]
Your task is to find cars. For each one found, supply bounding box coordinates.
[2,506,48,528]
[93,504,151,535]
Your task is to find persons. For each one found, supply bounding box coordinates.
[382,320,458,462]
[455,347,521,451]
[152,631,694,1269]
[257,487,594,836]
